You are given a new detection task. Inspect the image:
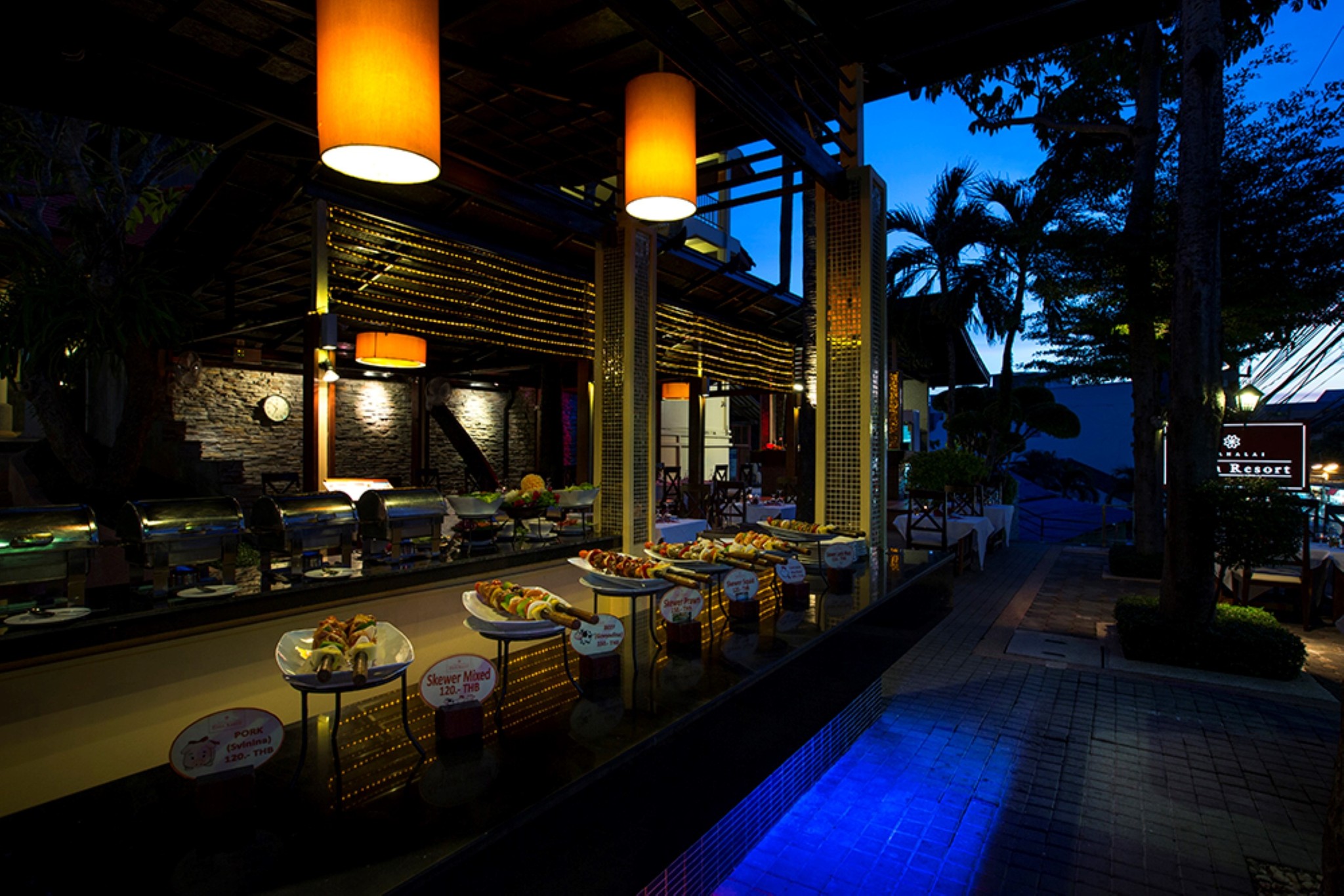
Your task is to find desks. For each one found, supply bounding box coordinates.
[721,502,798,528]
[655,519,712,544]
[939,502,1014,547]
[1296,535,1344,572]
[892,513,994,571]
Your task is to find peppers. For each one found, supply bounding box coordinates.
[645,541,733,563]
[474,579,551,618]
[319,615,376,652]
[742,517,819,547]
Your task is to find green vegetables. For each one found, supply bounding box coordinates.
[461,483,602,534]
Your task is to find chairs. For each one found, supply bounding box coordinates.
[661,465,748,523]
[1216,500,1344,632]
[905,484,1003,575]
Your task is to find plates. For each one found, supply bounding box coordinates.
[462,585,571,629]
[567,553,665,585]
[304,567,352,577]
[4,608,91,624]
[275,621,415,688]
[177,585,242,598]
[756,520,840,539]
[643,543,726,562]
[788,535,868,566]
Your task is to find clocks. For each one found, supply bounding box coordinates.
[262,394,290,423]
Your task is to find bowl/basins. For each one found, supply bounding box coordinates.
[552,489,599,505]
[446,496,506,514]
[529,523,553,535]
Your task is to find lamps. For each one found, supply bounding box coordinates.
[662,383,690,402]
[355,329,428,370]
[316,0,440,185]
[624,52,698,222]
